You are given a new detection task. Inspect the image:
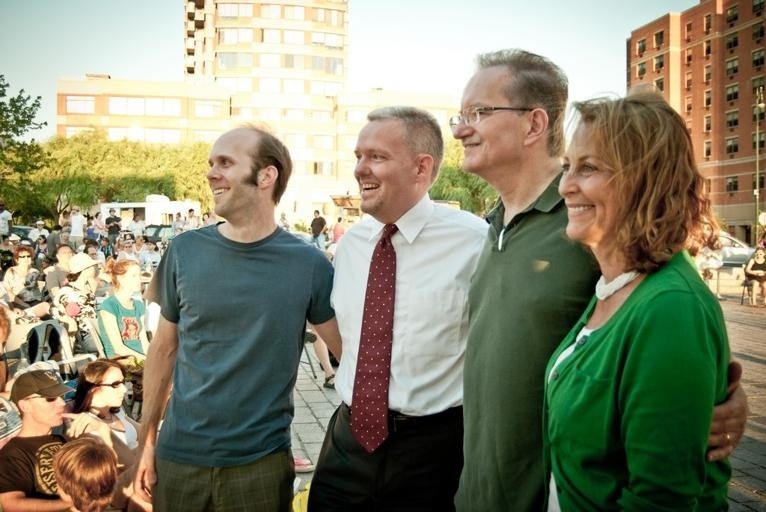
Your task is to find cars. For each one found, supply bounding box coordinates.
[715,232,758,266]
[289,230,331,249]
[8,223,34,240]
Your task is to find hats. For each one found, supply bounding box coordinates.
[68,253,100,274]
[150,241,160,251]
[10,367,73,404]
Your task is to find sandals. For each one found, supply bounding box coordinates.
[322,373,335,389]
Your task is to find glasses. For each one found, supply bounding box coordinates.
[16,392,66,403]
[18,254,33,259]
[446,103,542,129]
[88,377,128,390]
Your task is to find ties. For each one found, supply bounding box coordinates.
[348,224,399,453]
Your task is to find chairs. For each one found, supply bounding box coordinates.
[739,271,761,306]
[0,280,152,454]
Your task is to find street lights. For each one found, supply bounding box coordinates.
[752,82,766,254]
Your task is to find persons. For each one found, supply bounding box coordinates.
[306,104,492,512]
[540,88,736,511]
[305,208,344,389]
[748,242,766,310]
[109,122,340,509]
[448,46,751,511]
[0,202,208,510]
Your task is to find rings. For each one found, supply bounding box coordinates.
[725,432,731,445]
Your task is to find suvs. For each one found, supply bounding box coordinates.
[138,221,174,242]
